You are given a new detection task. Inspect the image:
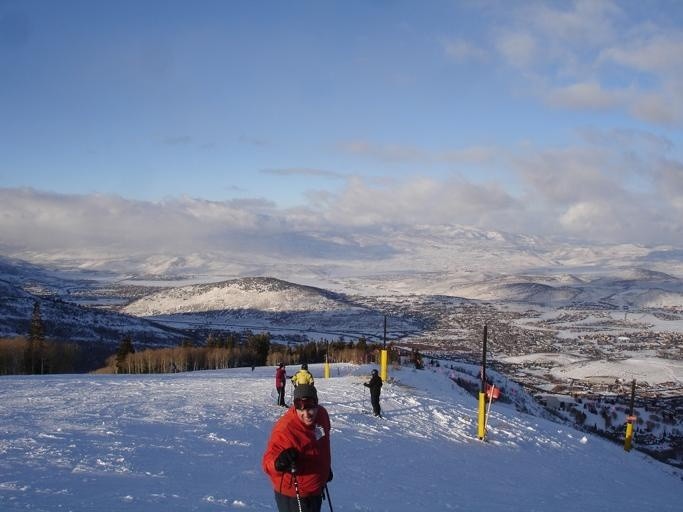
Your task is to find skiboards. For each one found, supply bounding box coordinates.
[361,410,391,421]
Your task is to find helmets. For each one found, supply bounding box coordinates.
[370,369,378,375]
[301,363,308,369]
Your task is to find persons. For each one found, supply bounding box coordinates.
[262,385,333,512]
[363,370,385,418]
[275,364,314,407]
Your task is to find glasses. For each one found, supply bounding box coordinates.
[294,399,315,409]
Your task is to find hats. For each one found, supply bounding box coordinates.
[279,362,285,369]
[293,384,318,403]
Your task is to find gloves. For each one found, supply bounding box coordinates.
[274,448,299,474]
